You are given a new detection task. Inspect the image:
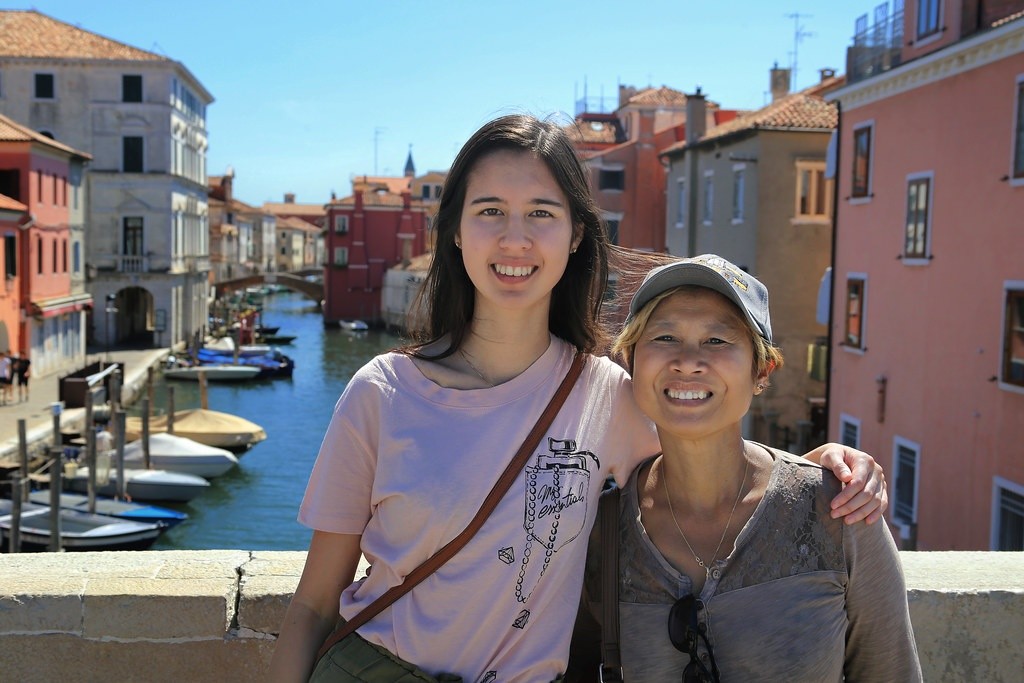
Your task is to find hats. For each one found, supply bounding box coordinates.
[623,254,773,353]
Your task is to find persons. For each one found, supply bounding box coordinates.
[0,345,34,405]
[565,253,924,683]
[272,112,891,682]
[212,315,242,338]
[239,313,254,347]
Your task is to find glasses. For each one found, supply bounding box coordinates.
[668,597,720,683]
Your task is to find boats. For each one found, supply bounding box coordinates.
[27,487,188,535]
[77,432,241,481]
[159,282,301,378]
[73,468,210,505]
[0,499,172,552]
[125,407,267,457]
[337,319,370,331]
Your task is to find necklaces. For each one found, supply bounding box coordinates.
[458,347,497,389]
[659,452,751,581]
